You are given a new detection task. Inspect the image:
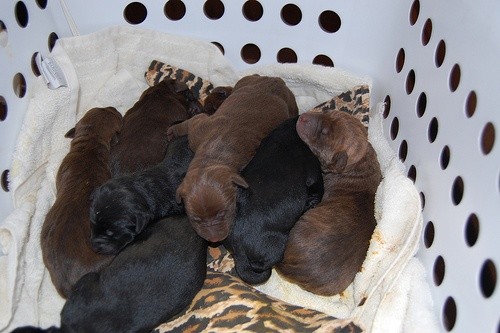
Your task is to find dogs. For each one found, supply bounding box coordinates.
[106,78,204,179]
[87,133,196,256]
[40,106,123,300]
[273,109,383,296]
[8,213,208,333]
[223,115,324,284]
[175,73,299,244]
